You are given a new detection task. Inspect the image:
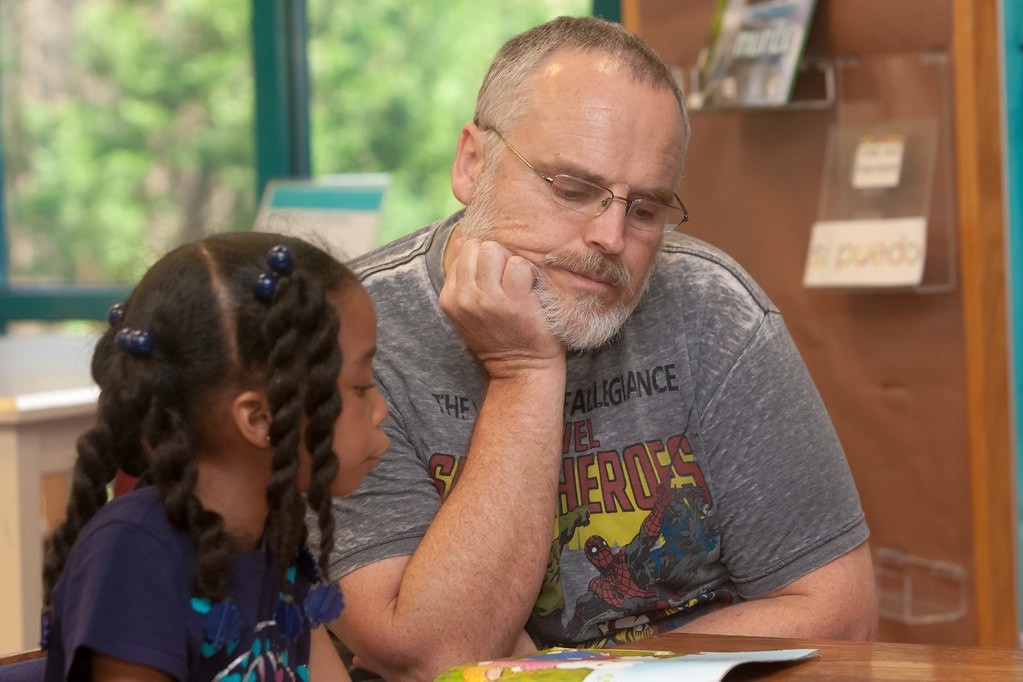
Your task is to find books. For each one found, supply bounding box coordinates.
[699,0,817,105]
[433,648,819,682]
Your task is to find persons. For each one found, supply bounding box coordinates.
[304,15,877,682]
[41,231,392,682]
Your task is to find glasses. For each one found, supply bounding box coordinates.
[476,118,689,233]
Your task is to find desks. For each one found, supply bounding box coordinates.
[433,632,1023,682]
